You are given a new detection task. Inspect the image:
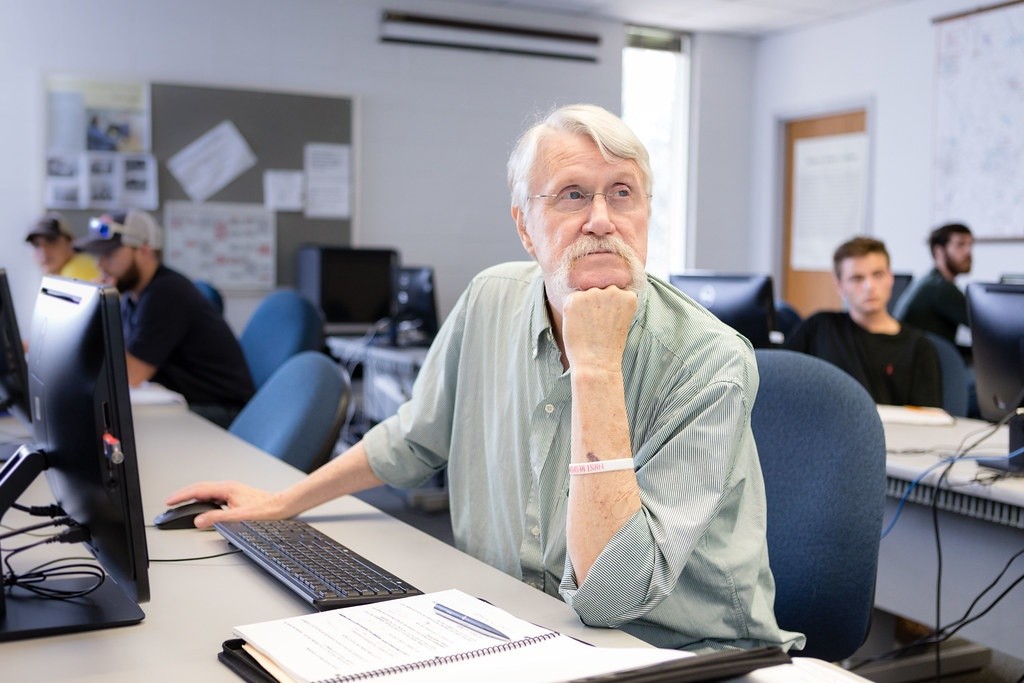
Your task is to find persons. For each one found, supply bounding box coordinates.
[792,238,944,410]
[894,224,980,418]
[167,105,807,659]
[22,217,104,355]
[72,208,257,430]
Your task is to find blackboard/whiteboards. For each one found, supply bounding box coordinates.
[927,1,1024,245]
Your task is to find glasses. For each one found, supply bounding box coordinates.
[526,188,652,212]
[87,215,143,240]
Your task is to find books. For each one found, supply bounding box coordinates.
[231,589,697,683]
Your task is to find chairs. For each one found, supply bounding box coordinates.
[238,290,328,392]
[752,348,886,662]
[226,351,351,474]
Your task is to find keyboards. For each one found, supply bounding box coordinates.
[212,520,424,614]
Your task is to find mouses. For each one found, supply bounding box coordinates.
[154,502,223,531]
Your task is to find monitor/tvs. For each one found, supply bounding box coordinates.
[669,274,783,350]
[0,268,33,426]
[0,273,152,640]
[296,243,440,348]
[963,282,1024,475]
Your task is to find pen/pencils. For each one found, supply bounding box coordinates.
[434,604,513,642]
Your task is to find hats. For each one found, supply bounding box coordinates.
[25,217,74,242]
[74,210,163,253]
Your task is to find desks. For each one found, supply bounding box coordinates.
[329,332,1024,663]
[0,351,659,683]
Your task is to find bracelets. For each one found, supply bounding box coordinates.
[568,459,635,476]
